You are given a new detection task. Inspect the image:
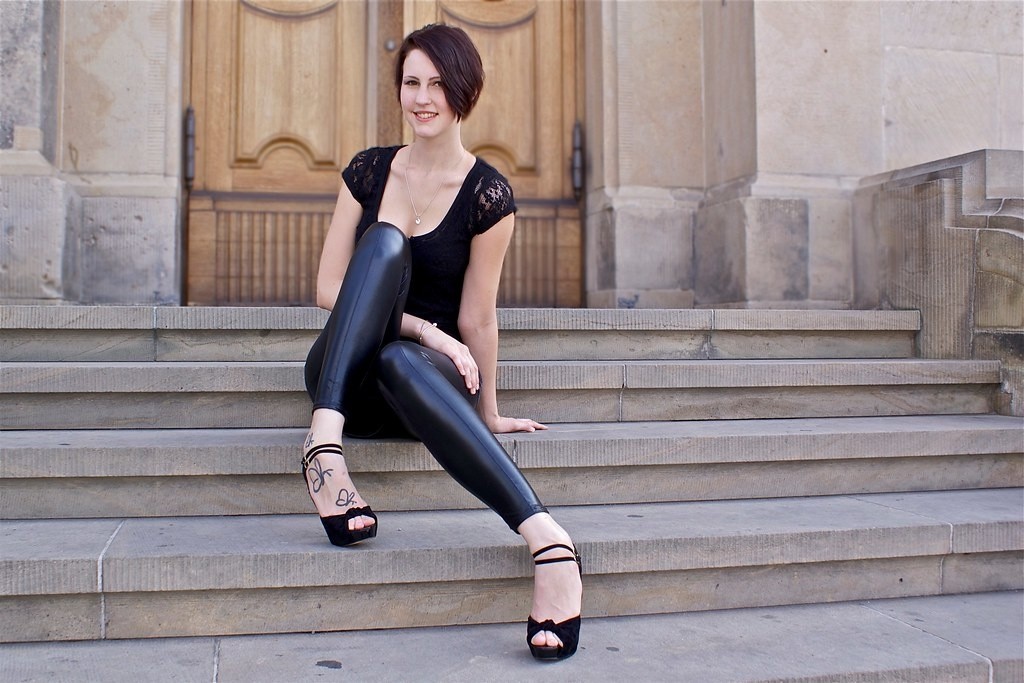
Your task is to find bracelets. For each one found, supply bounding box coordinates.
[417,320,429,339]
[419,323,438,346]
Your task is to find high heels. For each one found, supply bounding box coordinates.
[301,444,379,547]
[526,543,583,662]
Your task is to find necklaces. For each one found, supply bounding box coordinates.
[404,143,465,224]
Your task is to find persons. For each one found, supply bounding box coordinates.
[302,25,583,662]
